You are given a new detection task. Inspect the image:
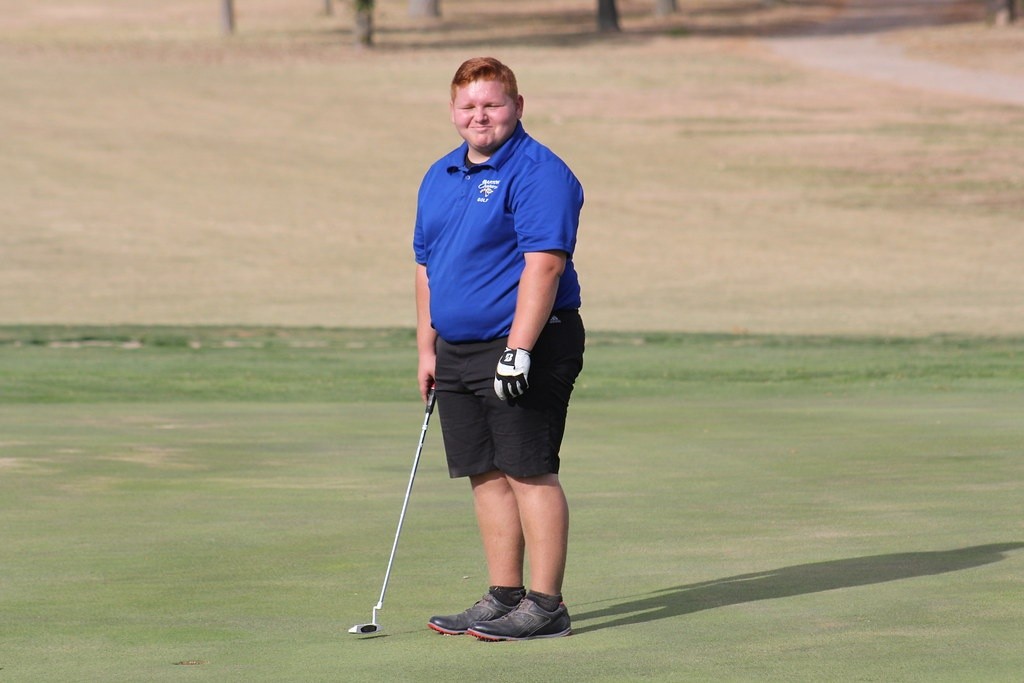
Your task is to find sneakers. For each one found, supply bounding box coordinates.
[469,598,573,641]
[428,592,527,637]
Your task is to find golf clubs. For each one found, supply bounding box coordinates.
[348,380,437,635]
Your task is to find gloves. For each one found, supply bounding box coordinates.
[493,347,532,402]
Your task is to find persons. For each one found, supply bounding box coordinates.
[412,58,586,640]
